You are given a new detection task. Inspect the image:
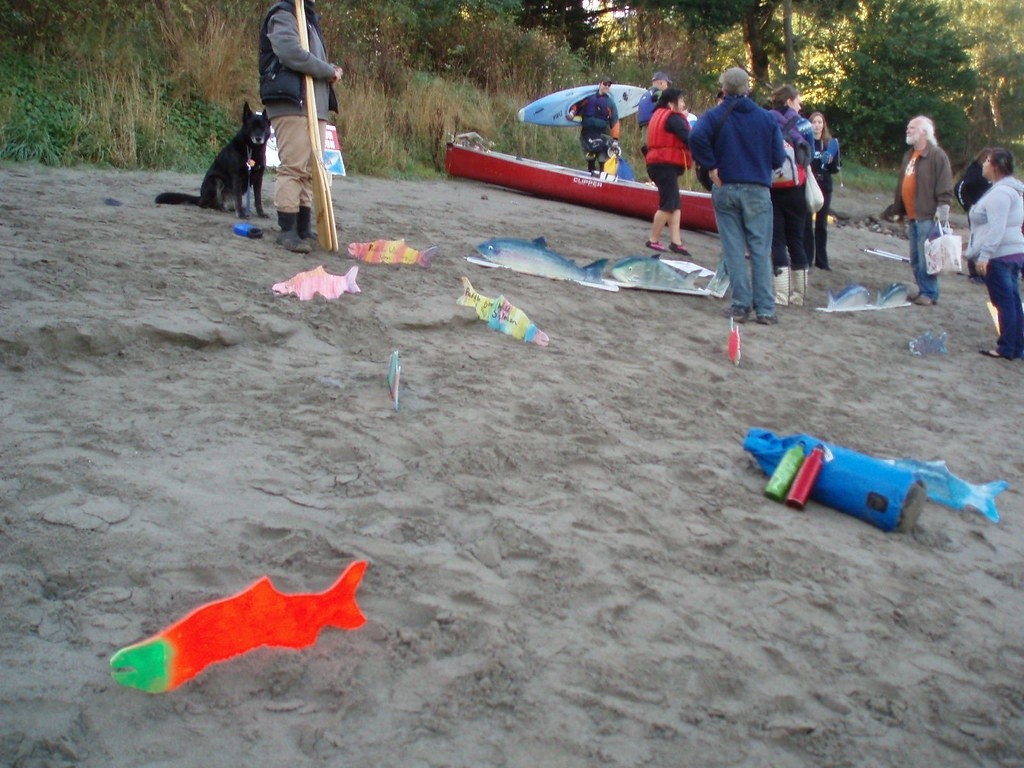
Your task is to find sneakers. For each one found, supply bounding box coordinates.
[646,240,667,251]
[669,241,691,256]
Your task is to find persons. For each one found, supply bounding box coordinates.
[258,0,343,254]
[637,72,691,257]
[894,116,954,305]
[954,146,1024,361]
[567,77,619,173]
[687,67,844,324]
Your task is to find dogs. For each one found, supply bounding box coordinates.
[154,101,272,220]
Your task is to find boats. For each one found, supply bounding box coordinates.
[443,130,720,233]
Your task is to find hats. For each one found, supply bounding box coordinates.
[600,76,613,84]
[653,73,672,84]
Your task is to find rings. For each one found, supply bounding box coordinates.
[980,268,983,271]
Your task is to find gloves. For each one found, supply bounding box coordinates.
[565,112,573,122]
[935,205,950,221]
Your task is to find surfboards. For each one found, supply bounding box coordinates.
[517,82,653,127]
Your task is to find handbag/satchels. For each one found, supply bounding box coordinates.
[696,160,711,191]
[804,164,826,212]
[924,212,962,275]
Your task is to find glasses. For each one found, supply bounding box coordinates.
[602,83,611,87]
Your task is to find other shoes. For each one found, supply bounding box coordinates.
[979,344,1012,360]
[757,315,778,325]
[729,304,748,323]
[907,290,937,305]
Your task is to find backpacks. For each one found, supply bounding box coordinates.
[771,116,807,189]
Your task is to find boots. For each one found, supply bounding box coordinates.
[790,269,810,306]
[278,210,312,253]
[772,266,789,306]
[298,204,318,241]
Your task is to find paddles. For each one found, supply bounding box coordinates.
[859,246,912,264]
[294,7,340,253]
[600,133,635,180]
